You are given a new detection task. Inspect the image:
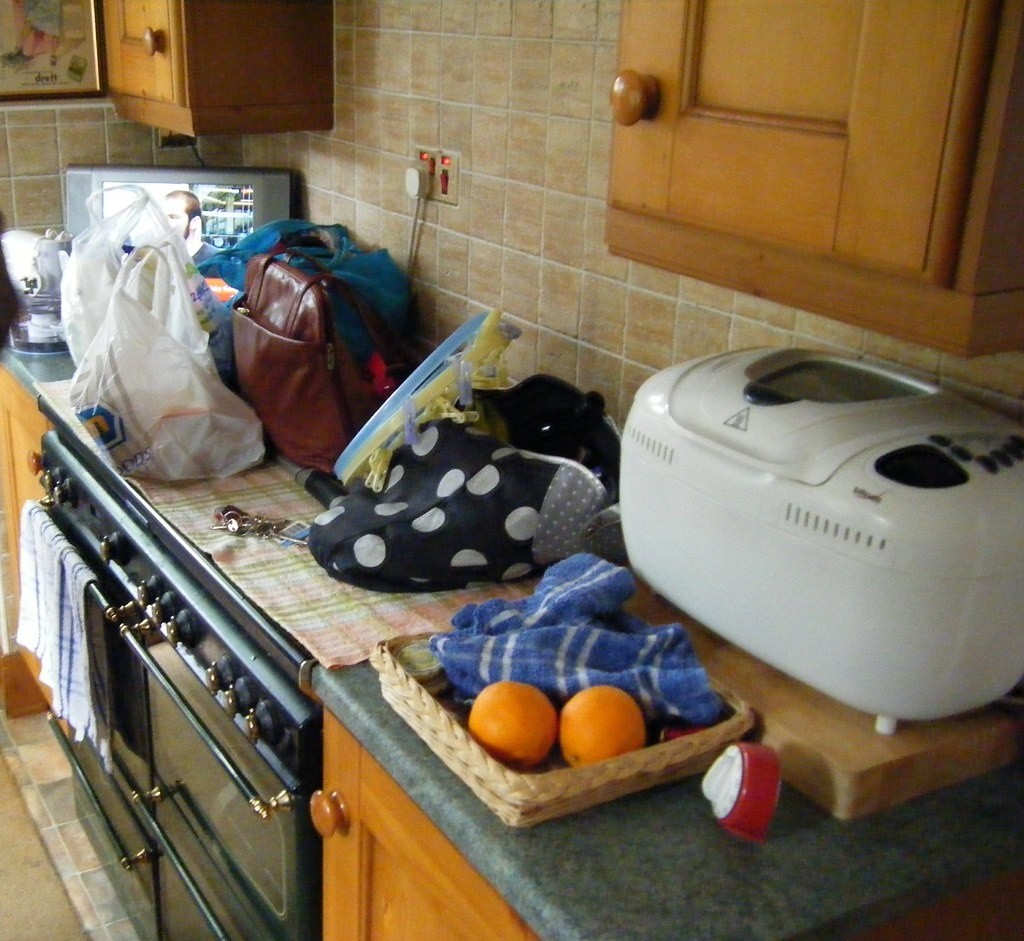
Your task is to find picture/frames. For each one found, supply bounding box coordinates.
[0,0,109,101]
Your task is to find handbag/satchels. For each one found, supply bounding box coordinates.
[309,374,623,593]
[57,183,266,481]
[230,243,429,474]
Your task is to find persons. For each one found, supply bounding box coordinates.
[161,186,223,278]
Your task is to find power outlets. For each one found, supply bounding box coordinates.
[412,146,437,200]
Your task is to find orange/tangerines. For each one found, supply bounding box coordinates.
[467,680,647,770]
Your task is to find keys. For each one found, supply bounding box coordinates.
[209,503,306,548]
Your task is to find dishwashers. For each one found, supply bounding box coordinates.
[18,396,322,941]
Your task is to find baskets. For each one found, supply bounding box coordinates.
[370,630,753,828]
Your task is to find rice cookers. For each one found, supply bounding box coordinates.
[618,341,1024,734]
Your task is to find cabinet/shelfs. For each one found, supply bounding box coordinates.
[96,0,334,138]
[0,336,79,739]
[308,668,1024,941]
[603,0,1024,360]
